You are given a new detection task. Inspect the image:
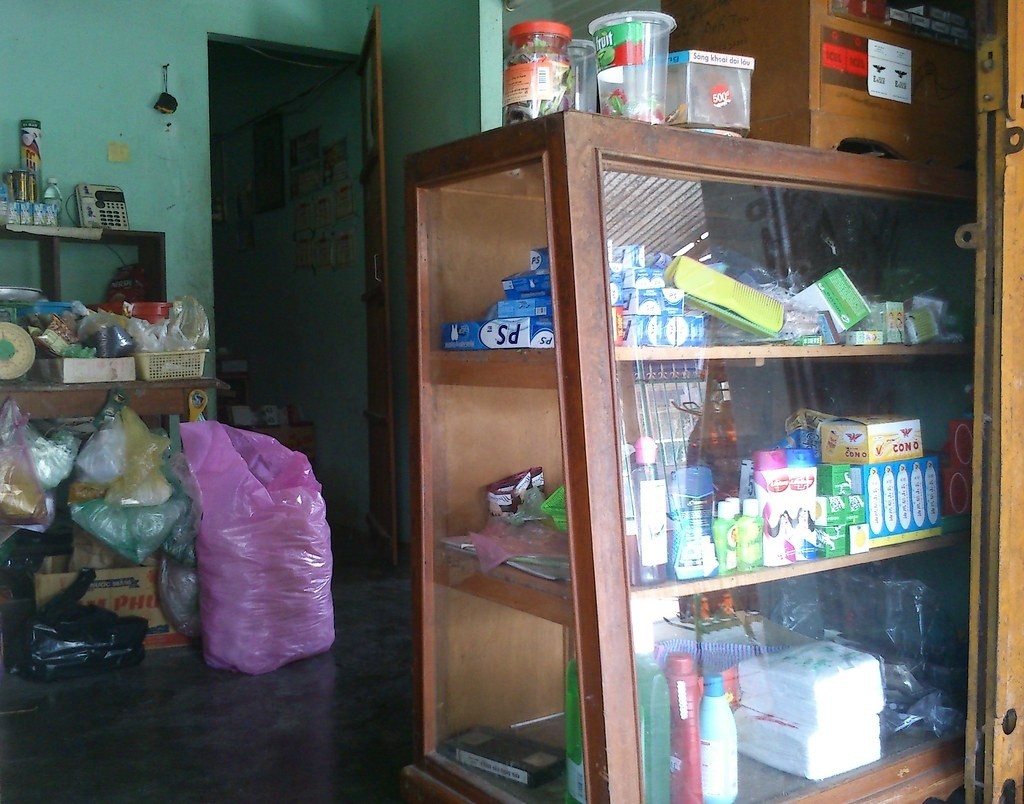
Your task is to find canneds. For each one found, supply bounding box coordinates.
[3,171,37,203]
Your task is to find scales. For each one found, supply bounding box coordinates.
[0,286,43,385]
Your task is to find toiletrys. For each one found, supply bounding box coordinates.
[624,436,817,586]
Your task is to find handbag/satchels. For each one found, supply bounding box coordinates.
[3,566,149,684]
[0,395,201,631]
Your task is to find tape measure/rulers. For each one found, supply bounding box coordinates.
[154,91,178,113]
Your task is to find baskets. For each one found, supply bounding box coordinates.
[135,349,207,384]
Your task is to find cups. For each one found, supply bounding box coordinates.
[589,11,677,125]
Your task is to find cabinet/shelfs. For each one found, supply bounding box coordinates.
[661,0,976,172]
[399,106,974,804]
[0,223,232,423]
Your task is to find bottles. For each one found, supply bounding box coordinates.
[632,435,819,582]
[44,177,64,227]
[562,645,739,803]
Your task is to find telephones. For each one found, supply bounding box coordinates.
[74,183,130,230]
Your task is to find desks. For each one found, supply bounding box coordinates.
[216,374,250,411]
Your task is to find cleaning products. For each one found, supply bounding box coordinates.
[567,646,736,804]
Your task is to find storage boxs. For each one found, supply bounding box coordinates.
[262,405,280,426]
[28,357,136,384]
[216,360,248,374]
[128,348,210,381]
[249,421,316,460]
[833,0,975,49]
[622,50,756,137]
[228,404,251,425]
[17,301,80,320]
[31,541,199,649]
[435,239,974,787]
